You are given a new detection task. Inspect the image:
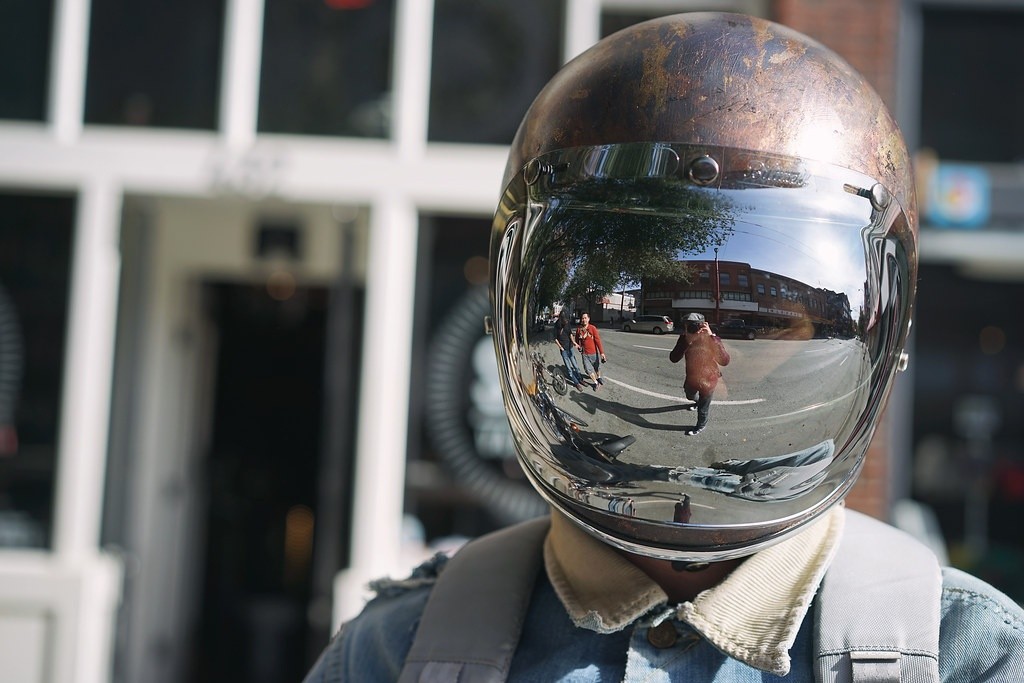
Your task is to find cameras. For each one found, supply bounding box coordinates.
[601,359,606,363]
[687,322,702,334]
[578,347,583,352]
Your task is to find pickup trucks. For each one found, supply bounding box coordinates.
[709,319,765,340]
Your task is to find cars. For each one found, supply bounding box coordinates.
[622,315,674,335]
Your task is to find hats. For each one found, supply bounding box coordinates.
[680,312,704,321]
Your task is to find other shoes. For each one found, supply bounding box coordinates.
[576,384,583,392]
[598,378,603,385]
[579,380,588,387]
[688,425,706,436]
[690,403,698,410]
[593,382,599,391]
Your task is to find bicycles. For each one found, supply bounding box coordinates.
[530,352,636,463]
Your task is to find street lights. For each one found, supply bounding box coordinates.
[714,247,720,324]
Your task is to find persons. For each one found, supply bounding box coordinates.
[669,314,730,435]
[301,11,1023,683]
[554,310,588,392]
[575,313,606,391]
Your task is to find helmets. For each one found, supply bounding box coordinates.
[485,12,920,566]
